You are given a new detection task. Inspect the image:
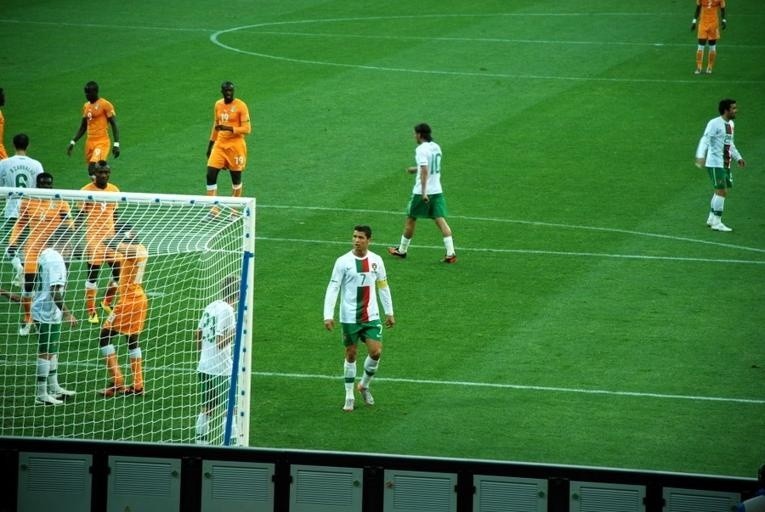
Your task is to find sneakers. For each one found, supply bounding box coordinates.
[19,321,31,337]
[49,388,77,399]
[34,394,63,408]
[200,212,221,222]
[357,383,375,406]
[388,247,406,259]
[101,301,114,315]
[707,220,732,232]
[343,399,355,412]
[98,383,126,397]
[88,312,99,325]
[119,385,143,397]
[440,253,456,263]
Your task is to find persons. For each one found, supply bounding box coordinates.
[67,81,121,182]
[27,223,77,406]
[388,123,458,263]
[72,162,122,324]
[690,1,727,74]
[194,276,241,447]
[0,89,8,159]
[7,172,75,337]
[694,99,746,232]
[206,82,251,224]
[0,133,45,261]
[97,224,149,396]
[323,227,395,413]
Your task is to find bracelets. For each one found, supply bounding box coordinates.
[692,18,697,24]
[113,142,120,148]
[722,19,726,23]
[69,140,76,146]
[123,231,130,239]
